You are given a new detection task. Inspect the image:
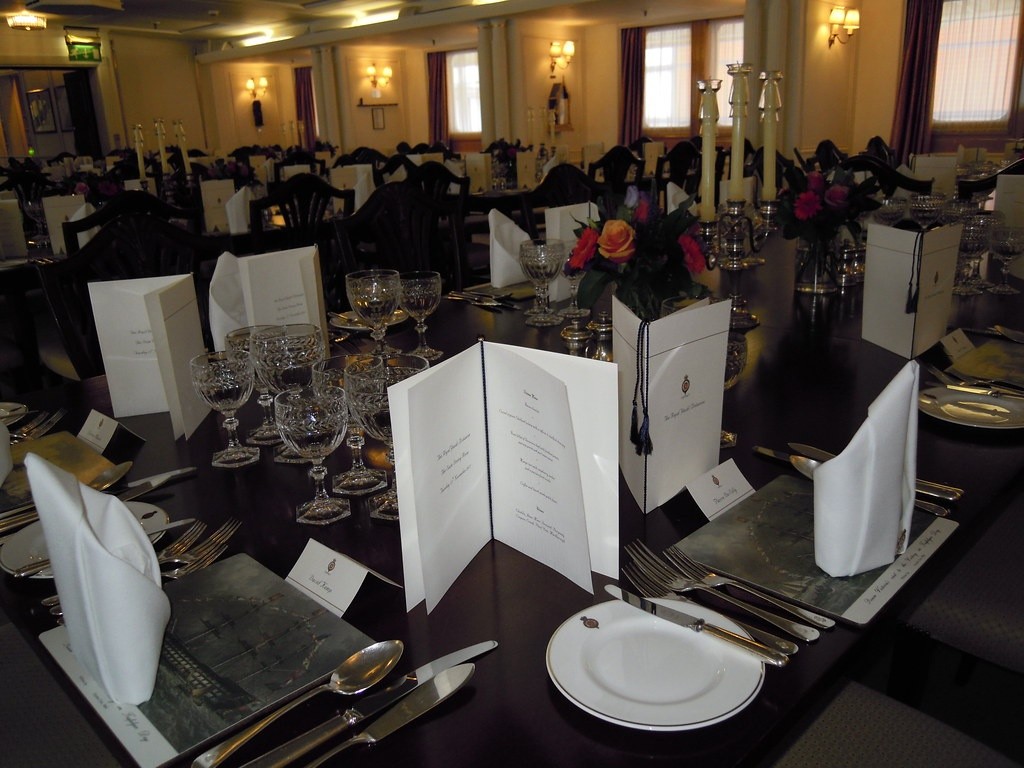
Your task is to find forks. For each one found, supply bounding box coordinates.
[0,404,75,450]
[927,362,1024,402]
[620,537,838,662]
[39,511,244,630]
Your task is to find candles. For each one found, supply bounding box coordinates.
[131,120,149,182]
[727,62,753,203]
[526,105,535,145]
[280,118,305,151]
[755,68,783,200]
[694,75,722,224]
[171,118,192,176]
[547,109,556,145]
[151,112,171,174]
[539,103,547,144]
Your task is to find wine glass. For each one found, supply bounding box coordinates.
[871,187,1023,303]
[186,260,458,529]
[516,238,593,326]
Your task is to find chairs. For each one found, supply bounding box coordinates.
[0,133,1023,768]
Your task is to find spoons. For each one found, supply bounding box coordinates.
[995,325,1024,344]
[790,455,949,520]
[461,290,514,301]
[1,461,134,532]
[188,639,403,768]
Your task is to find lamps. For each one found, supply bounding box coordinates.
[247,74,268,99]
[6,9,47,32]
[366,61,393,89]
[548,38,576,73]
[826,6,861,49]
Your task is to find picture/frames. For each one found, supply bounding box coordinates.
[371,107,386,130]
[26,88,57,134]
[51,85,75,132]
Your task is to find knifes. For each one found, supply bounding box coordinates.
[299,662,476,768]
[604,583,788,667]
[448,290,503,306]
[1,482,155,532]
[790,442,965,503]
[237,640,498,768]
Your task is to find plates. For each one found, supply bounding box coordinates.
[1,501,169,580]
[545,597,765,730]
[917,386,1024,432]
[0,402,28,429]
